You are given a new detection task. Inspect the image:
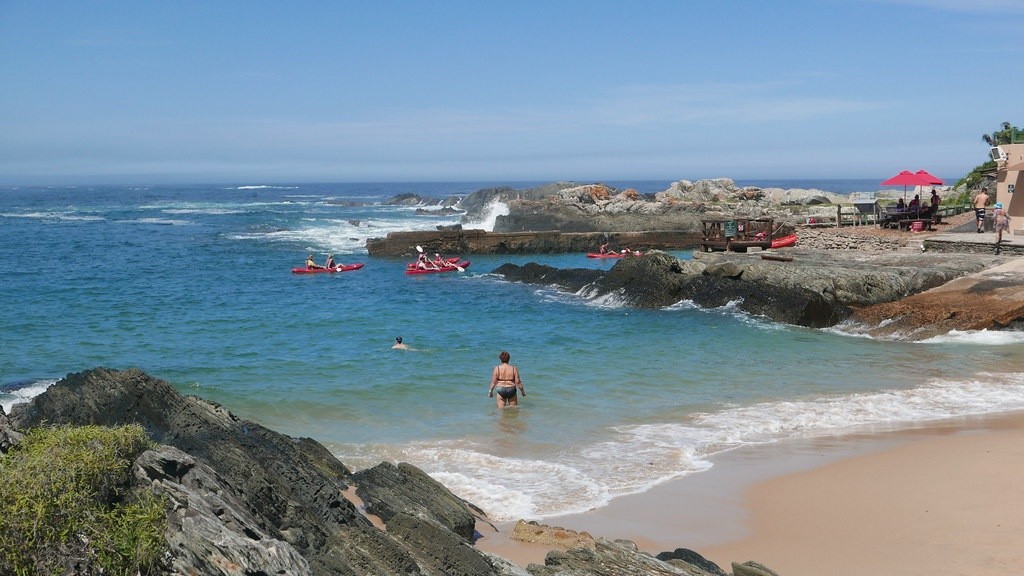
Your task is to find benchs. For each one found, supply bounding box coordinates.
[899,219,934,232]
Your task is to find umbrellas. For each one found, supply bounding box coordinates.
[881,169,944,227]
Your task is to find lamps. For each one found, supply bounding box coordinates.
[990,146,1008,162]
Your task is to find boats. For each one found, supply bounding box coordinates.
[587,251,644,258]
[405,260,471,274]
[407,257,461,268]
[292,262,365,272]
[769,234,798,248]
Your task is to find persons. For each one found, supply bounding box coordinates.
[973,187,989,233]
[392,336,409,350]
[326,255,346,269]
[896,189,941,231]
[993,201,1011,245]
[487,351,527,408]
[417,249,453,271]
[306,255,323,269]
[754,230,766,242]
[599,242,633,255]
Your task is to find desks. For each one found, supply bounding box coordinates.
[887,212,925,230]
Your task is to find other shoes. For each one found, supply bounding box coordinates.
[977,228,984,233]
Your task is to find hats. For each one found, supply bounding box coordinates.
[308,255,313,259]
[993,203,1002,209]
[930,189,936,194]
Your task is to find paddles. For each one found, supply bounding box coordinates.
[435,253,465,272]
[416,245,438,268]
[336,267,341,272]
